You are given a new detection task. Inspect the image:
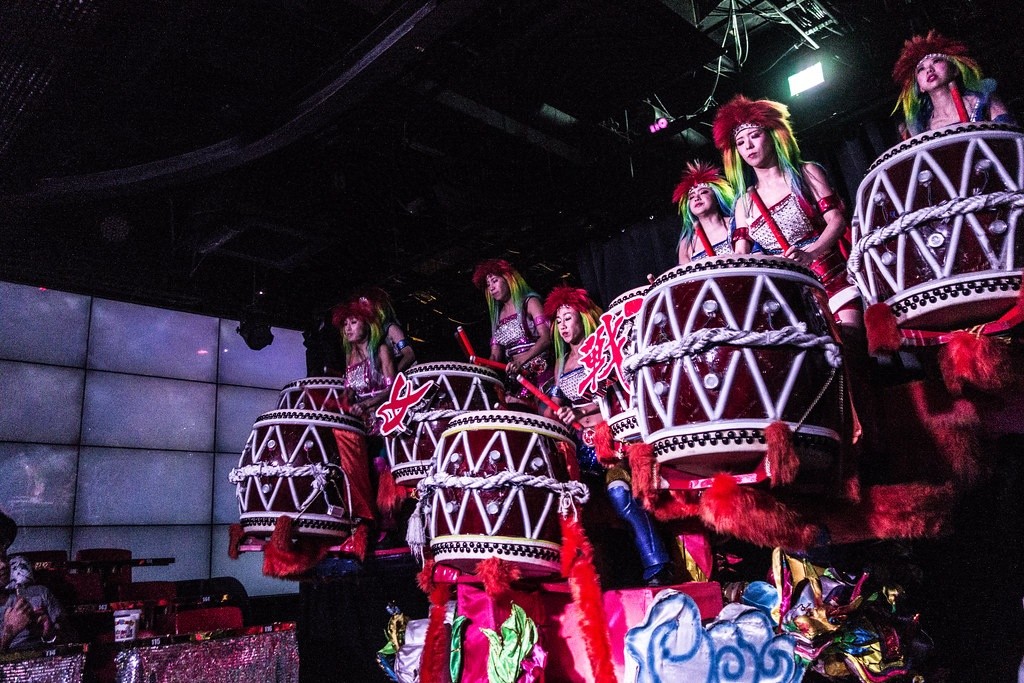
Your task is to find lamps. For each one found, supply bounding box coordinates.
[235,303,273,351]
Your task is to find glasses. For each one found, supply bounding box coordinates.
[0,537,10,547]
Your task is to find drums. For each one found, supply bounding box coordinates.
[428,408,577,579]
[634,254,847,474]
[845,120,1024,333]
[235,407,366,540]
[598,284,652,443]
[384,360,506,487]
[274,374,350,415]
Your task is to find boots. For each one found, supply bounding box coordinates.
[610,490,667,585]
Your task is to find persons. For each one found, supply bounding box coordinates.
[472,259,552,410]
[542,286,675,580]
[0,511,62,653]
[333,287,414,477]
[714,94,863,325]
[673,160,737,266]
[889,30,1008,141]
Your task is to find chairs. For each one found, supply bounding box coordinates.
[9,548,256,643]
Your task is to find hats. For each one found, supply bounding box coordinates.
[0,511,17,548]
